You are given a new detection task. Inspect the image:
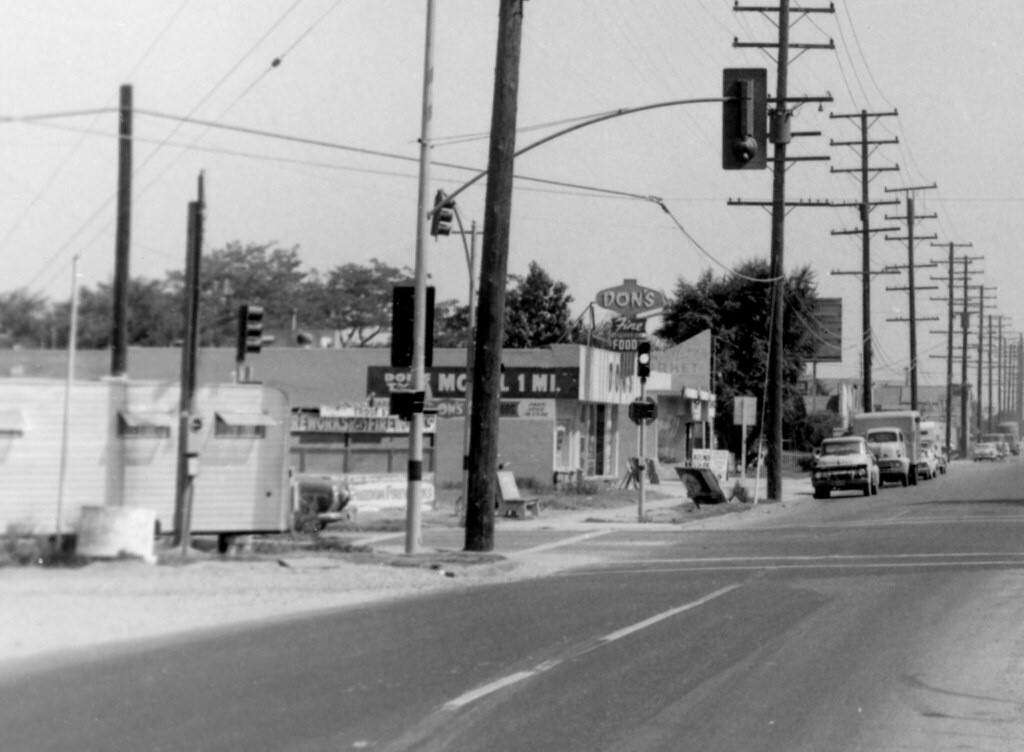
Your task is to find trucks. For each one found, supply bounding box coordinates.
[847,410,920,487]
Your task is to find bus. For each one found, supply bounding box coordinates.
[996,420,1018,440]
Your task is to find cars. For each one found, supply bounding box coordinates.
[973,432,1022,462]
[813,436,882,498]
[916,423,946,480]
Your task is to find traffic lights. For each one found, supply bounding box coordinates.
[431,191,455,235]
[245,305,263,352]
[636,340,651,378]
